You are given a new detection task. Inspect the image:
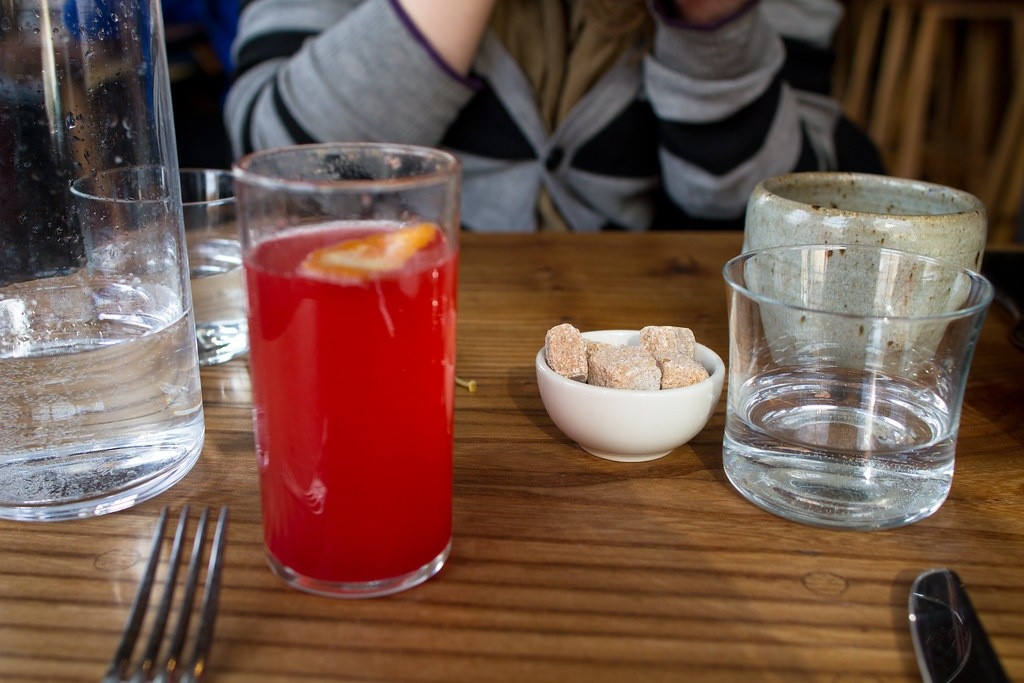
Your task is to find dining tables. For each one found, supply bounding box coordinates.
[0,232,1023,683]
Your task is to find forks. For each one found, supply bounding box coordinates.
[98,503,231,683]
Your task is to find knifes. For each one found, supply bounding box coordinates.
[906,565,1013,683]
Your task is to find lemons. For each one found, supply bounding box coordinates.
[301,224,437,281]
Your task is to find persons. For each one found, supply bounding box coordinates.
[225,1,819,232]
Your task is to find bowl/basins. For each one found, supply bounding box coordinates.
[536,331,726,462]
[742,171,987,383]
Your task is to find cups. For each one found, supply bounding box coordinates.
[66,163,253,370]
[229,142,462,599]
[0,0,207,521]
[723,244,996,531]
